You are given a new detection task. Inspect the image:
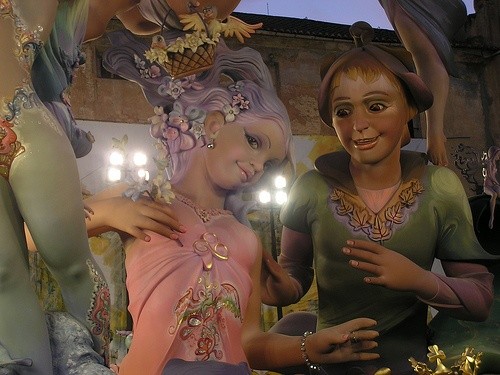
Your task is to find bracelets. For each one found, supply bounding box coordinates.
[302,331,320,370]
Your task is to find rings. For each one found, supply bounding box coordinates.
[351,332,357,344]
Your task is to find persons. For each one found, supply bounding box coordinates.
[0,0,365,375]
[481,145,500,230]
[260,21,494,374]
[378,1,460,166]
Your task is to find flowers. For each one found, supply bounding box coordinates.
[122,12,248,205]
